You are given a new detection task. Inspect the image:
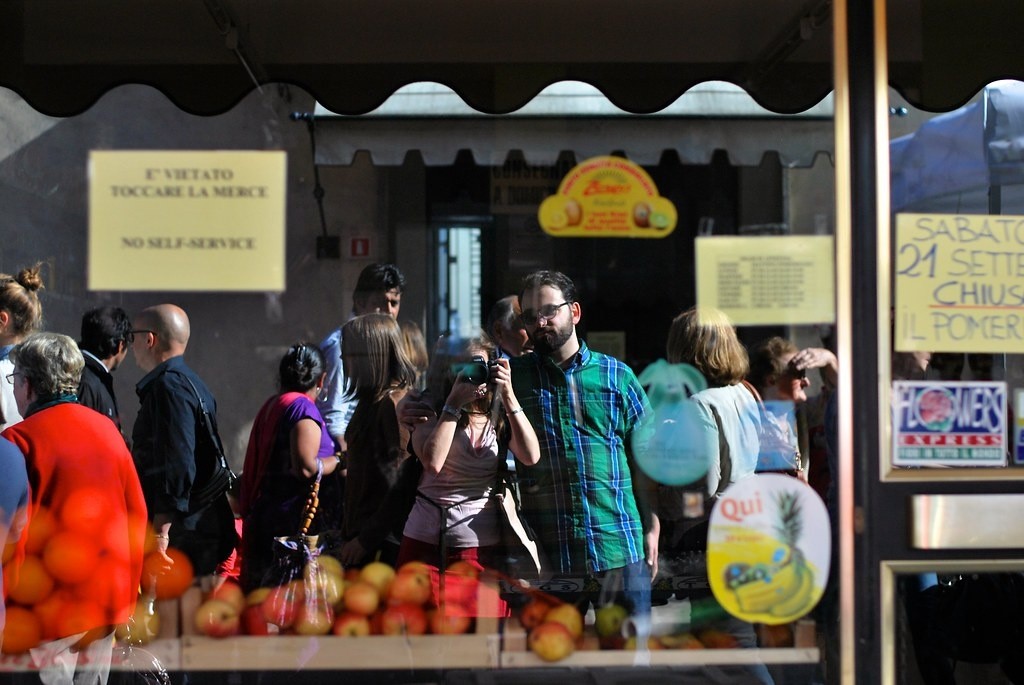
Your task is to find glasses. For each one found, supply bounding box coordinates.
[127,330,158,343]
[6,373,30,384]
[518,301,573,325]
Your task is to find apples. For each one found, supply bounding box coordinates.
[194,559,734,662]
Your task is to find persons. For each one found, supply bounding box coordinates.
[1,259,956,685]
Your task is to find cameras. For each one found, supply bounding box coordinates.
[458,355,499,386]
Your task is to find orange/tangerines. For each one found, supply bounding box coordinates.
[0,491,190,649]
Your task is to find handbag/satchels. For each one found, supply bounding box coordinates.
[261,456,342,588]
[493,418,540,573]
[177,451,237,515]
